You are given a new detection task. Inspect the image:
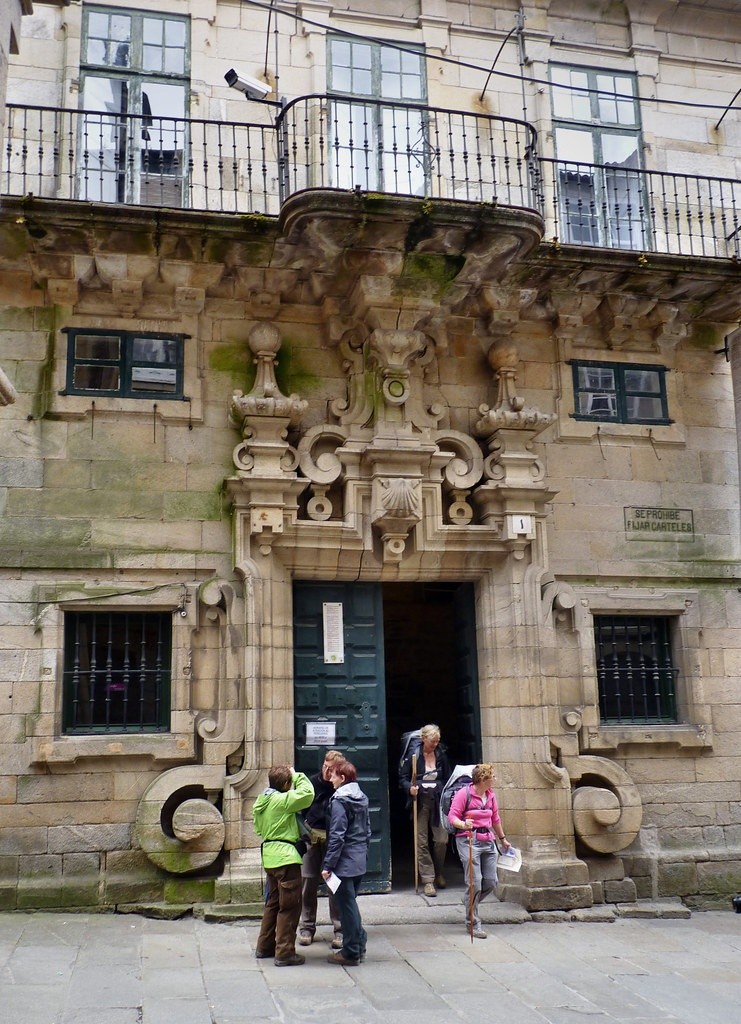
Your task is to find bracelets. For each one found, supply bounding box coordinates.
[498,835,507,839]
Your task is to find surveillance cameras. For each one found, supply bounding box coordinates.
[224,68,272,100]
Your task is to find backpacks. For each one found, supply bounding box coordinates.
[398,730,421,775]
[439,765,477,834]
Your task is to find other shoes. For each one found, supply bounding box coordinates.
[273,954,306,966]
[467,928,487,938]
[331,935,343,948]
[255,950,273,957]
[300,931,312,944]
[327,949,366,965]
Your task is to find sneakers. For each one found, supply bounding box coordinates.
[424,882,436,896]
[436,875,446,888]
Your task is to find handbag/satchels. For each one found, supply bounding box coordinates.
[263,839,307,857]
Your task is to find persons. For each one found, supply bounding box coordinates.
[400,725,449,897]
[448,764,511,939]
[298,750,370,966]
[253,763,315,966]
